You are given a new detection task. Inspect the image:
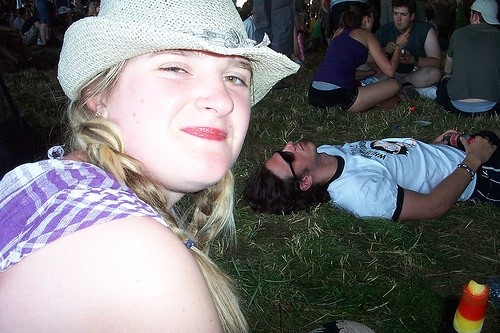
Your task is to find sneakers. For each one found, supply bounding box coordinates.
[414,86,441,100]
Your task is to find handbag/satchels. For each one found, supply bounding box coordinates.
[0,115,35,153]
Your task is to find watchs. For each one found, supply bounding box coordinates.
[409,56,419,66]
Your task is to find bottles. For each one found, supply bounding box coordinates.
[313,20,321,37]
[453,274,490,333]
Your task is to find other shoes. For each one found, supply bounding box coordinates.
[274,81,292,89]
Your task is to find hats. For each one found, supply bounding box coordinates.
[57,0,300,108]
[470,0,500,25]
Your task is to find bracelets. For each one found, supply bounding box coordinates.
[457,164,475,179]
[396,43,404,50]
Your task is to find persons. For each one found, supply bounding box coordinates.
[0,0,500,333]
[243,129,500,222]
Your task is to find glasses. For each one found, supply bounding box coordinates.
[274,148,298,181]
